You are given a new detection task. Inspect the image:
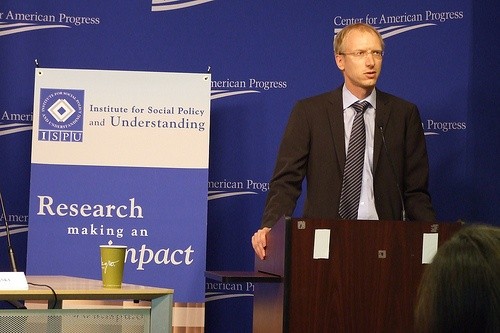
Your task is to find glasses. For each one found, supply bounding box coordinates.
[339,49,385,59]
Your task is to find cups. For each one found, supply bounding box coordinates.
[99,245,127,288]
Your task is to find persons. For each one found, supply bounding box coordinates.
[414,223,500,333]
[251,22,438,261]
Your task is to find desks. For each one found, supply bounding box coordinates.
[0,275,174,333]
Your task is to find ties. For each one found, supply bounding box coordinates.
[337,100,371,221]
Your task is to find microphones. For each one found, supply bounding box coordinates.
[376,118,406,221]
